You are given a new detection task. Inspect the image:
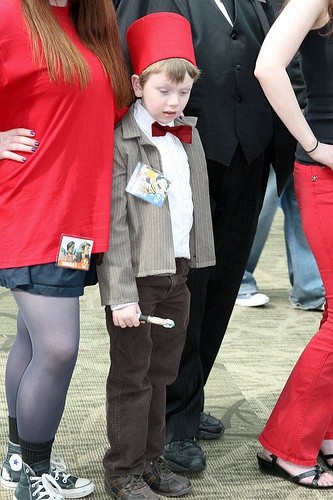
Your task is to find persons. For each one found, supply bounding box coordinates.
[235,164,327,312]
[116,0,307,471]
[253,0,333,488]
[96,12,216,500]
[0,0,135,499]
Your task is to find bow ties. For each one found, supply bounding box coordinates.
[151,121,193,143]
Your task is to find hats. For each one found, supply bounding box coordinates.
[127,12,196,76]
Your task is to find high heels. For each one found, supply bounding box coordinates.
[315,448,333,474]
[256,447,333,489]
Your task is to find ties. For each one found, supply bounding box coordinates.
[219,0,236,25]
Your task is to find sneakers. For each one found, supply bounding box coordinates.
[1,440,96,498]
[234,292,270,306]
[104,473,161,500]
[316,300,327,310]
[142,457,194,496]
[12,458,66,500]
[196,411,225,439]
[159,436,206,471]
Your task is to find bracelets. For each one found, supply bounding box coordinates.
[304,140,318,153]
